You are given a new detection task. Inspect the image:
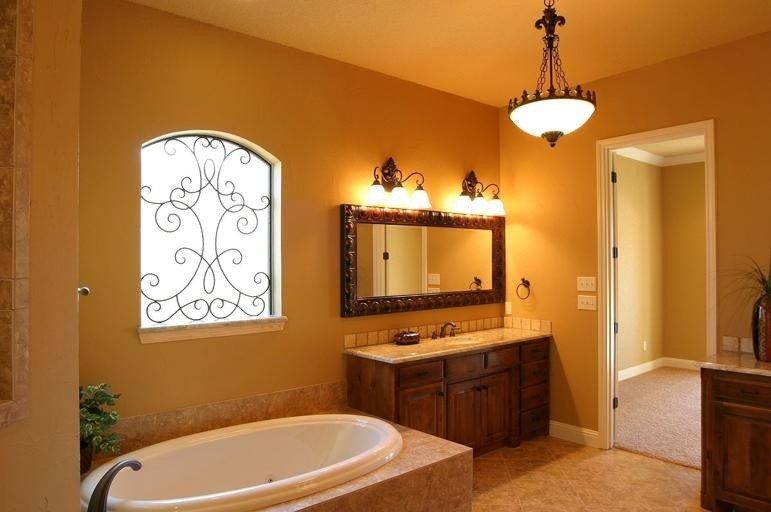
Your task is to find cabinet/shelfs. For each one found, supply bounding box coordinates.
[521,334,553,442]
[442,340,522,458]
[343,348,447,440]
[700,363,771,512]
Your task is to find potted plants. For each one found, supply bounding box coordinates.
[79,379,126,475]
[712,255,771,363]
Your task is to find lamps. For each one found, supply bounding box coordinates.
[362,156,434,214]
[449,168,507,219]
[506,1,601,153]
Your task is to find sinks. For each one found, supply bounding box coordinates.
[444,336,482,345]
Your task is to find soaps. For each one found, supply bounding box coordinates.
[395,330,423,343]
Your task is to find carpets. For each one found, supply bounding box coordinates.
[614,366,704,469]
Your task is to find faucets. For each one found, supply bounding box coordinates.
[438,320,458,338]
[88,458,142,512]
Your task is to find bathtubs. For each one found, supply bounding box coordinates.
[80,412,404,511]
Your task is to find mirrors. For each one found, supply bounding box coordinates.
[339,203,508,319]
